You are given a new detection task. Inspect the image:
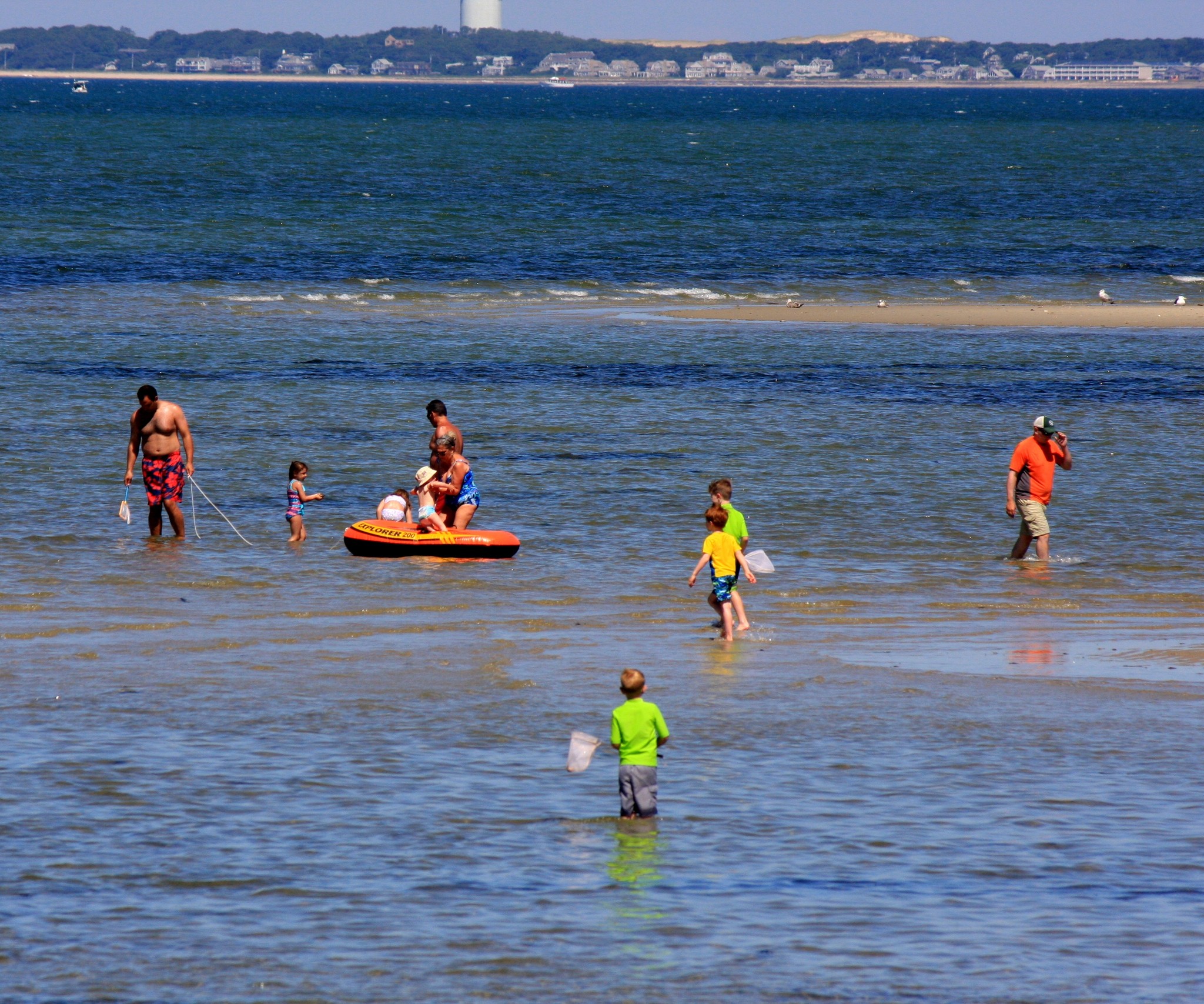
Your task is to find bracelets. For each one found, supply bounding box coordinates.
[438,487,442,495]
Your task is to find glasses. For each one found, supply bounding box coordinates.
[1039,429,1054,436]
[427,410,433,420]
[435,447,451,457]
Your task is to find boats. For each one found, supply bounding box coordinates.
[343,518,521,559]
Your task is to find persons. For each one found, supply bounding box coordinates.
[425,399,463,513]
[377,489,413,524]
[410,466,453,533]
[688,505,756,641]
[708,479,749,632]
[609,669,669,821]
[286,461,325,542]
[1007,416,1072,560]
[435,431,479,530]
[124,385,194,539]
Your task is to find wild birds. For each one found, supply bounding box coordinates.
[1099,289,1115,305]
[786,298,804,308]
[877,299,889,308]
[1174,296,1187,306]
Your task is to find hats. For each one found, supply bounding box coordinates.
[1033,416,1057,433]
[414,466,437,486]
